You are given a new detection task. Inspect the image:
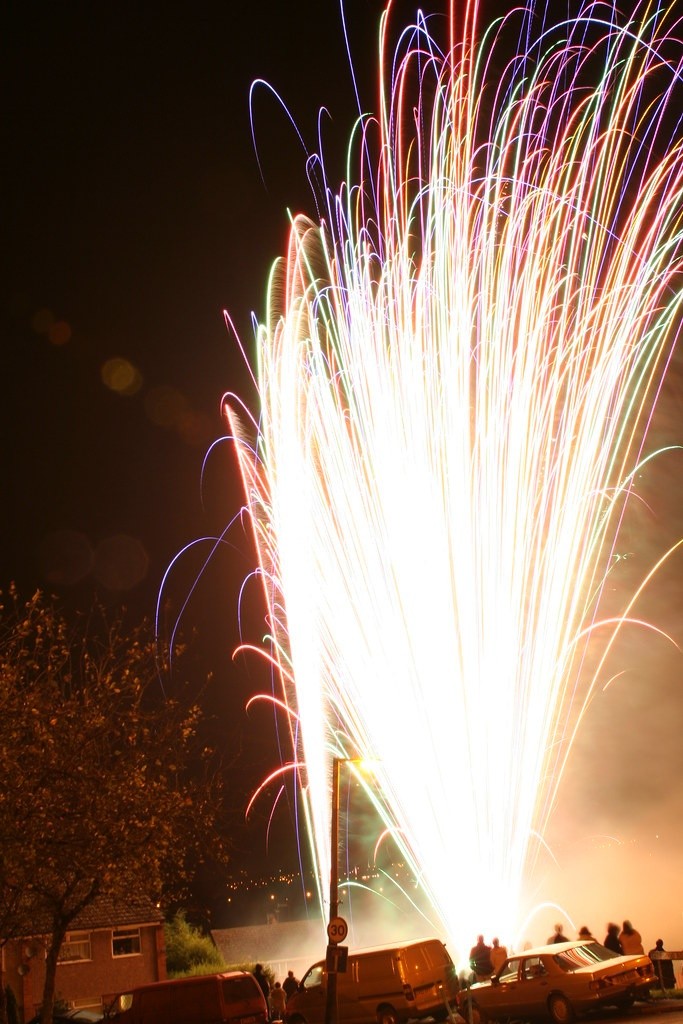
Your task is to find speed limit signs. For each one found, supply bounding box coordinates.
[326,917,349,944]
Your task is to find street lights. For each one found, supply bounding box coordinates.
[320,755,367,1024]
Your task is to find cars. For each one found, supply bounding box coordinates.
[453,939,659,1024]
[27,1006,102,1024]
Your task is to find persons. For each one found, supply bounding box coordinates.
[551,919,645,959]
[648,940,676,990]
[468,935,511,982]
[253,964,301,1020]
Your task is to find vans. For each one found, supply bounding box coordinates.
[283,938,461,1024]
[101,969,268,1024]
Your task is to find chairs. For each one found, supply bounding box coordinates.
[530,964,544,976]
